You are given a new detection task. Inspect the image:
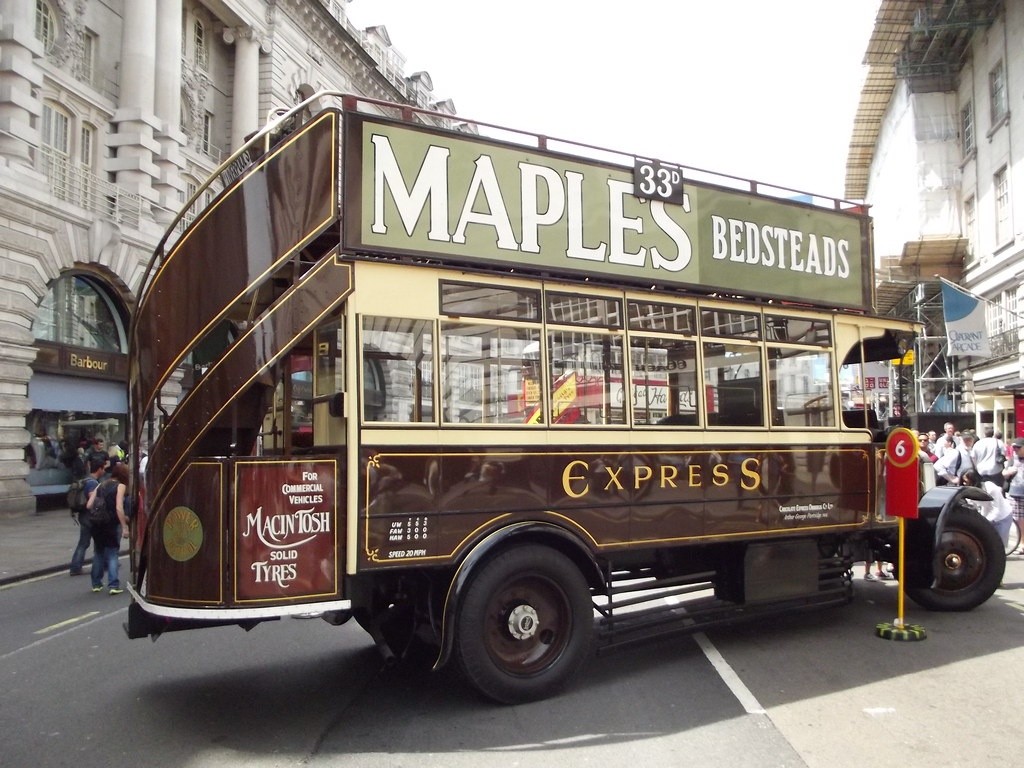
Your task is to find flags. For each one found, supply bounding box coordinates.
[940,280,993,357]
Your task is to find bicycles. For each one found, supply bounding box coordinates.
[1002,516,1023,555]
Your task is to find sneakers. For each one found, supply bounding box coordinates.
[108,587,124,595]
[92,585,103,591]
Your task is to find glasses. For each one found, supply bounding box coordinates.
[1013,445,1022,449]
[918,438,928,443]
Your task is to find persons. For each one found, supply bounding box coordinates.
[958,467,1014,590]
[1001,437,1024,556]
[971,429,1007,499]
[754,446,789,525]
[477,458,507,488]
[86,464,129,594]
[995,431,1004,441]
[25,433,74,468]
[1006,431,1015,457]
[86,438,129,486]
[935,422,960,459]
[933,429,981,488]
[360,457,402,513]
[69,457,105,576]
[75,447,88,479]
[423,456,440,500]
[911,428,938,463]
[863,559,893,582]
[926,430,937,455]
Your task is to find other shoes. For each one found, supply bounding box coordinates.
[70,570,82,575]
[1013,546,1024,555]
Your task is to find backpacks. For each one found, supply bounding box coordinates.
[87,480,120,527]
[66,477,95,527]
[933,448,962,487]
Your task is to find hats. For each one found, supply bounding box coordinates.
[1012,436,1024,447]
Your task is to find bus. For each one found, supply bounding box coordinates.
[121,90,1010,706]
